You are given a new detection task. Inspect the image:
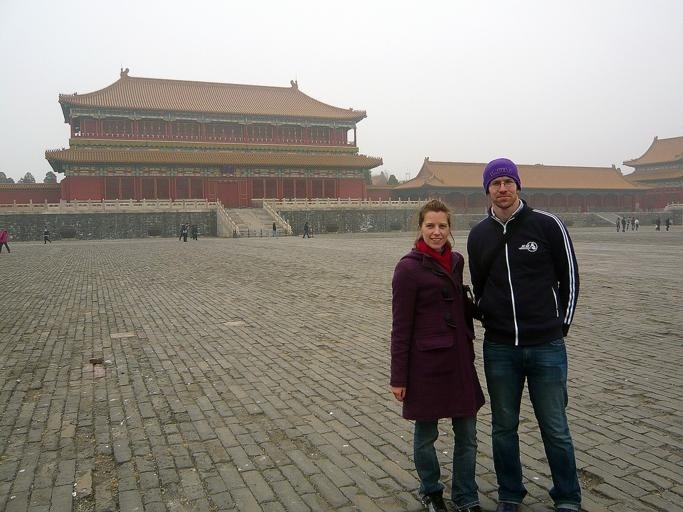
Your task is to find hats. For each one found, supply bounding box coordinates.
[482,158,521,195]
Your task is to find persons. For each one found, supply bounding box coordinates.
[303,222,310,238]
[43,226,51,244]
[0,227,10,253]
[390,199,485,512]
[468,157,582,512]
[656,217,661,232]
[273,222,276,237]
[665,218,671,231]
[616,216,640,232]
[179,223,198,242]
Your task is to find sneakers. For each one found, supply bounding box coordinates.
[551,503,580,512]
[421,490,447,512]
[497,502,517,512]
[450,500,482,512]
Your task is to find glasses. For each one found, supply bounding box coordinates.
[491,179,516,190]
